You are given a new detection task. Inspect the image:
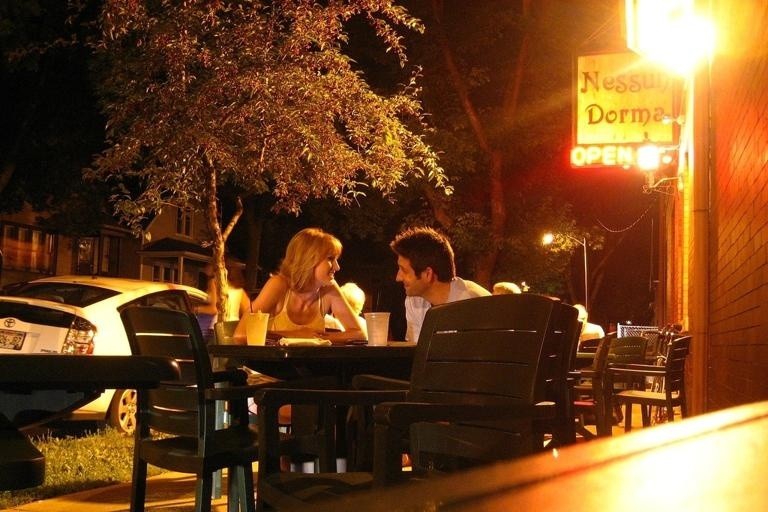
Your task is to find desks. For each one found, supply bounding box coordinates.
[203,337,426,512]
[278,397,767,512]
[0,352,170,494]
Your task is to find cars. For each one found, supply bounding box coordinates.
[0,275,217,438]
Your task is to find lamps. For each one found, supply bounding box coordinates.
[662,113,687,127]
[642,165,683,198]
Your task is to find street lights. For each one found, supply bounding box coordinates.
[543,232,589,315]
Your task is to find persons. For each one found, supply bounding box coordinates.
[492,281,519,297]
[231,228,369,427]
[571,302,611,371]
[388,225,499,345]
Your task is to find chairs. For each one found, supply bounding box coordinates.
[338,287,563,495]
[115,302,346,512]
[567,320,692,451]
[408,294,582,479]
[218,362,342,511]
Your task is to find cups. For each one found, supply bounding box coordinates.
[364,311,391,346]
[244,312,269,346]
